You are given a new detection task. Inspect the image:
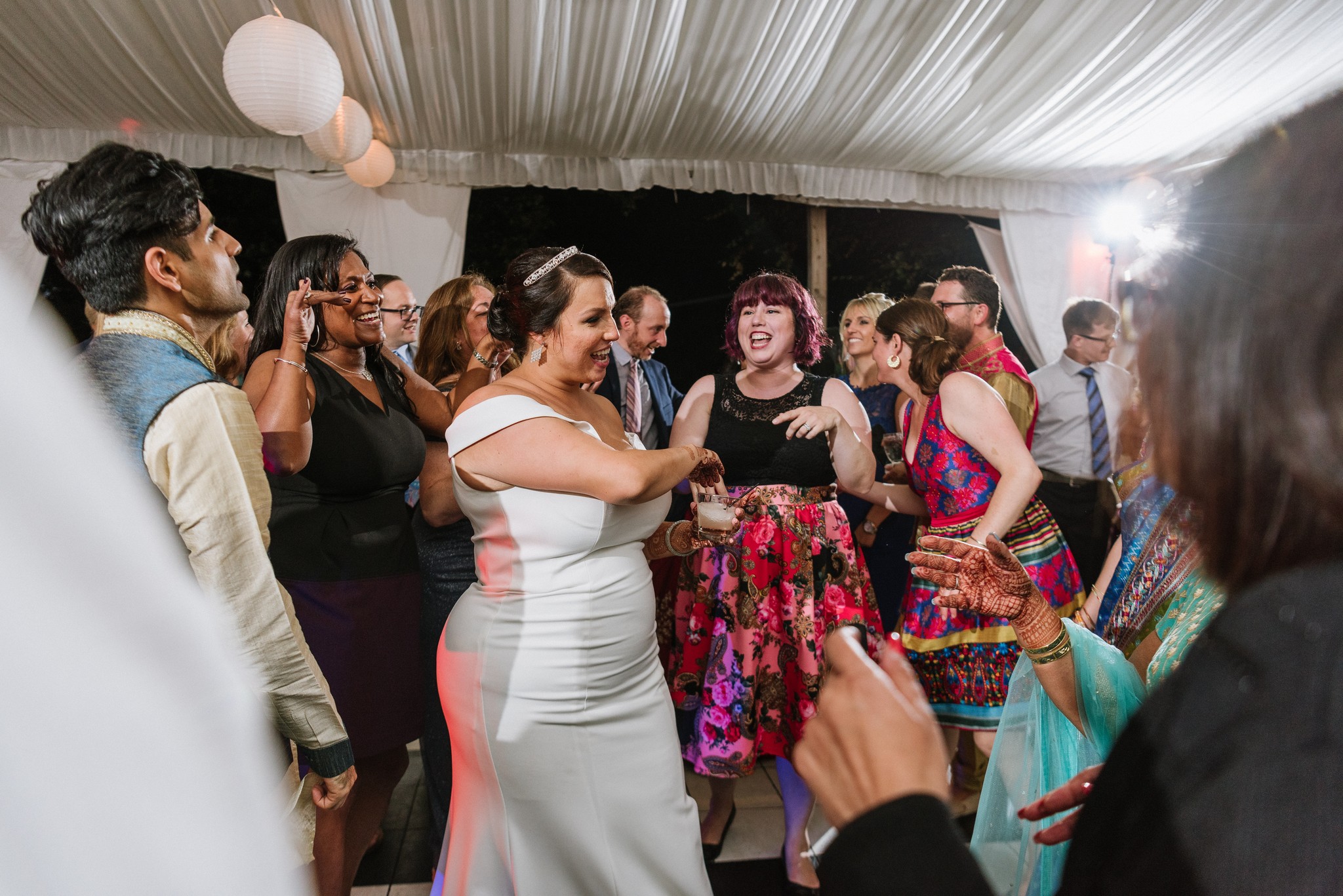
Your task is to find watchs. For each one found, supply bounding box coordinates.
[862,519,878,534]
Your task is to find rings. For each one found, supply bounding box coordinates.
[946,576,958,591]
[731,529,741,535]
[803,424,811,431]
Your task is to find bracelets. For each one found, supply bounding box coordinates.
[1090,584,1104,603]
[272,357,308,377]
[472,347,498,368]
[666,520,696,556]
[1028,636,1072,665]
[1072,606,1096,634]
[969,535,987,548]
[1021,616,1066,654]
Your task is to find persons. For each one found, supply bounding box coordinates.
[1073,428,1208,660]
[902,516,1232,896]
[793,95,1343,896]
[667,267,1086,896]
[23,144,722,896]
[1026,300,1142,600]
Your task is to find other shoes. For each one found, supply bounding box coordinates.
[701,802,736,863]
[779,845,823,896]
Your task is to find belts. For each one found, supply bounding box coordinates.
[1041,470,1092,487]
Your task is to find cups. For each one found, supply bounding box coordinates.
[696,491,742,543]
[883,432,904,465]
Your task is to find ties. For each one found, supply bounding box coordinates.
[1079,368,1112,480]
[626,357,641,433]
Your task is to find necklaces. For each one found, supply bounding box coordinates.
[311,349,374,382]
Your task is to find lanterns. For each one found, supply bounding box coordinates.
[223,16,394,188]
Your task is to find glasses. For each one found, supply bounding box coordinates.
[936,301,980,311]
[1080,334,1116,345]
[381,305,424,321]
